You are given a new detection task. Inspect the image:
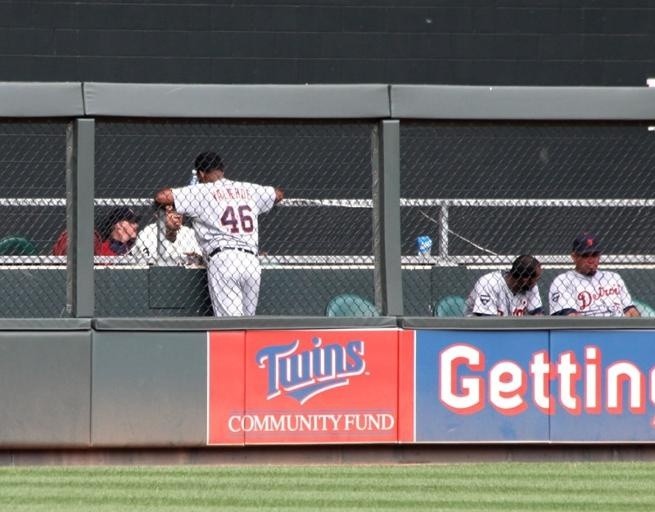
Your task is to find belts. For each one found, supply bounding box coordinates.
[208,247,257,261]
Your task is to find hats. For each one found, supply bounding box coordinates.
[109,207,141,224]
[194,151,223,170]
[572,234,600,255]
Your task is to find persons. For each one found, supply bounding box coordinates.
[127,200,200,265]
[155,152,283,317]
[95,205,143,256]
[548,233,642,317]
[462,255,545,316]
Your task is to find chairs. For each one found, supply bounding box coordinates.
[50,228,101,256]
[324,294,379,318]
[632,298,655,319]
[433,294,467,316]
[0,235,39,256]
